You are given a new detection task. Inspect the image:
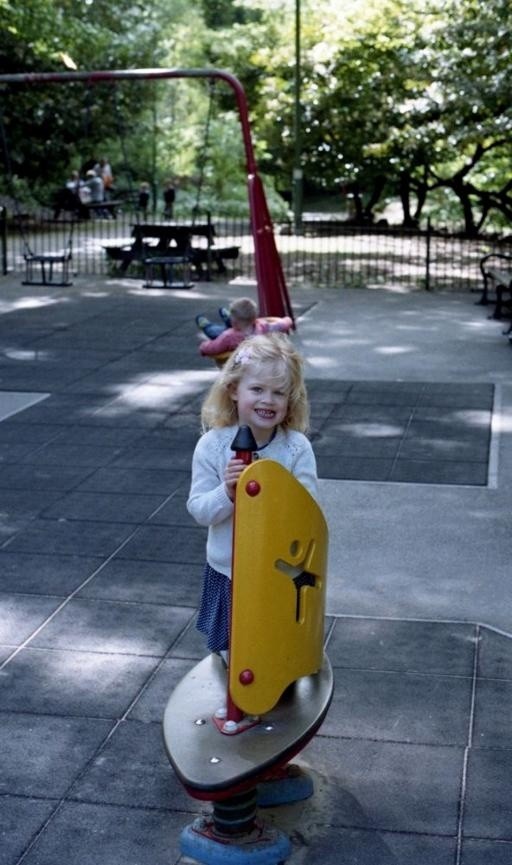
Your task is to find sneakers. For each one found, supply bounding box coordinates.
[196,315,211,330]
[219,307,231,322]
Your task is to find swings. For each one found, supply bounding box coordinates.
[111,76,218,291]
[0,78,91,287]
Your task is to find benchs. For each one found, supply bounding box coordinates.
[103,222,239,280]
[475,252,512,346]
[78,199,123,220]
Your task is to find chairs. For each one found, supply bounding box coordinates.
[14,219,195,290]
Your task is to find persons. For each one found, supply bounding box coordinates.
[93,157,114,219]
[194,298,294,356]
[65,169,84,193]
[137,182,150,222]
[162,178,176,222]
[77,169,106,220]
[186,331,320,673]
